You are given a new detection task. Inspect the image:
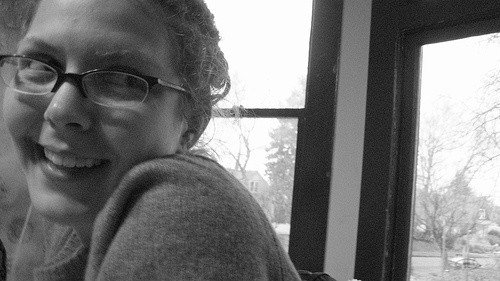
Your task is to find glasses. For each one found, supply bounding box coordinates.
[0,53,192,111]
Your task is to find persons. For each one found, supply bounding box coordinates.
[0,0,303,281]
